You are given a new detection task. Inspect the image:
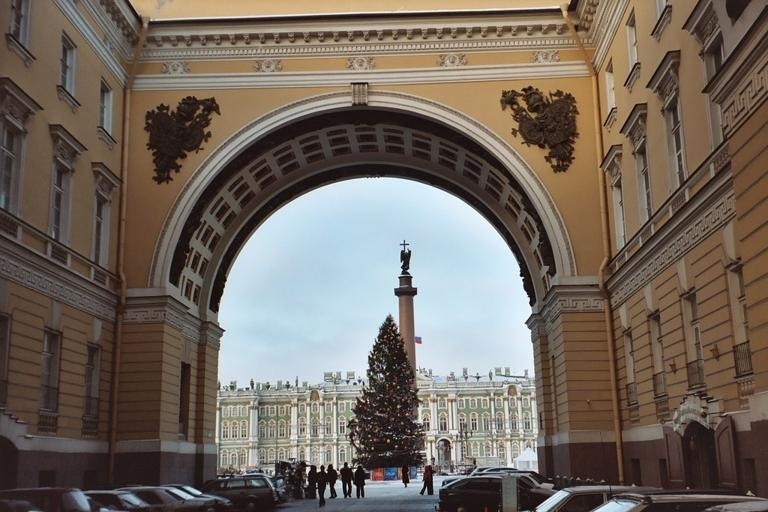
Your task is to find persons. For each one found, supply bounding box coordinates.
[295,462,364,508]
[420,462,436,495]
[402,463,409,488]
[428,458,439,493]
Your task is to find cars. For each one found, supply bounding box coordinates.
[0,474,287,512]
